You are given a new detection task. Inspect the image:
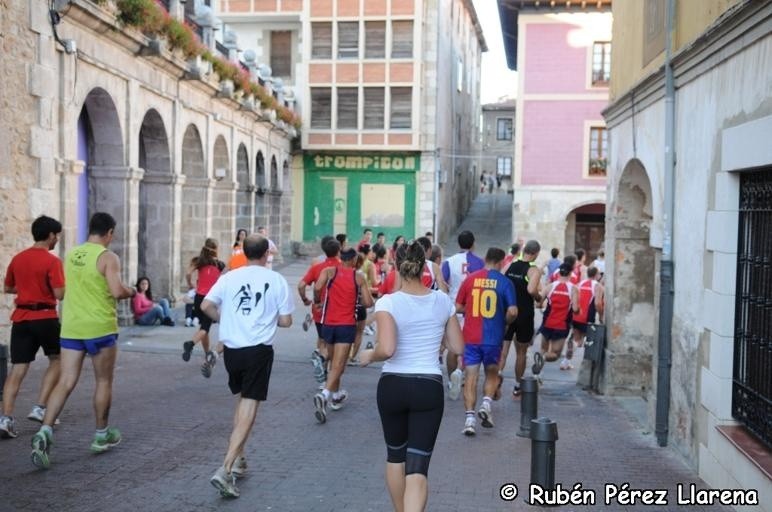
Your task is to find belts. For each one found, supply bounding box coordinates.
[16,303,57,311]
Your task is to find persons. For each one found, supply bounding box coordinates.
[131,229,605,512]
[0,216,65,438]
[30,213,136,469]
[480,170,502,194]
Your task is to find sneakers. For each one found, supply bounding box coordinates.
[311,348,348,425]
[346,356,365,368]
[30,431,52,472]
[27,406,62,426]
[90,427,122,453]
[230,456,250,479]
[0,415,19,438]
[364,326,375,336]
[512,351,574,398]
[201,352,217,378]
[448,360,503,437]
[159,316,199,327]
[183,341,195,361]
[303,314,312,331]
[210,466,242,499]
